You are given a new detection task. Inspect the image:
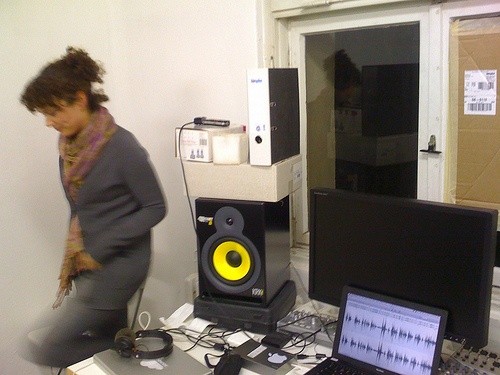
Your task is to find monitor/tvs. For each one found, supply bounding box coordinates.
[307,186,499,349]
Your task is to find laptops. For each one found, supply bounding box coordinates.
[303,285,449,375]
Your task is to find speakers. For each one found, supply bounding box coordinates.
[193,197,292,306]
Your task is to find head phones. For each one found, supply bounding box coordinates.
[114,326,174,360]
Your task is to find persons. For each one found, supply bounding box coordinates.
[12,47,166,368]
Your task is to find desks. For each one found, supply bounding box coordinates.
[69,300,331,375]
